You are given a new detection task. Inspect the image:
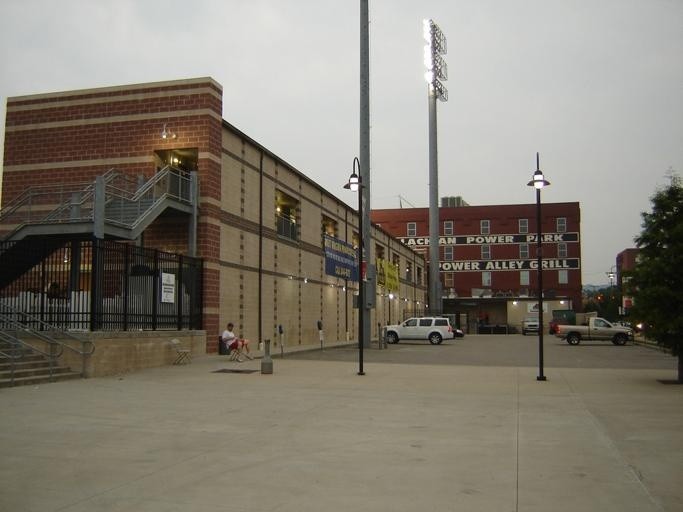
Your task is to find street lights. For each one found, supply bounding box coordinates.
[344,157,370,378]
[527,152,552,382]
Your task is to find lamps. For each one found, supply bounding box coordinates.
[161,121,176,140]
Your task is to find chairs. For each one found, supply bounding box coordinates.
[220,339,253,363]
[168,336,191,363]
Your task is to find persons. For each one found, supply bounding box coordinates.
[221,323,249,363]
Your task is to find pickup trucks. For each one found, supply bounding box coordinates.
[555,316,634,345]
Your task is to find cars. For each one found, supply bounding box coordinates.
[521,315,540,335]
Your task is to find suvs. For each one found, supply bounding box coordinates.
[380,316,454,345]
